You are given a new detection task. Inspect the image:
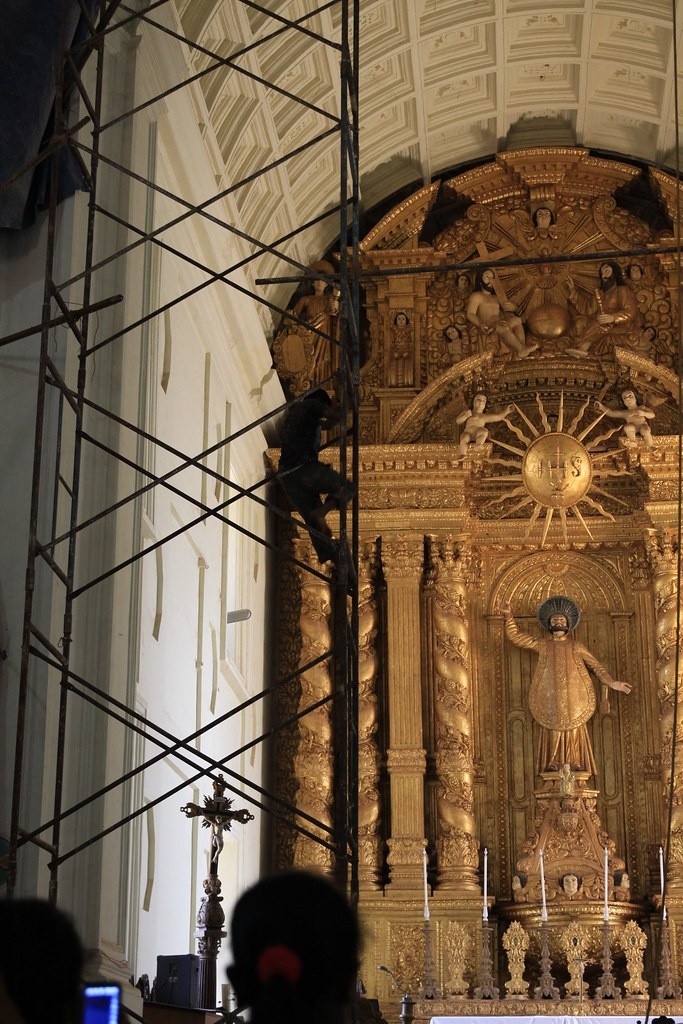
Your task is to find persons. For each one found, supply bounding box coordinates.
[443,326,463,363]
[532,207,555,228]
[593,389,655,447]
[226,871,389,1024]
[512,873,527,891]
[547,414,559,431]
[563,262,638,359]
[0,897,89,1023]
[496,598,634,772]
[635,326,657,358]
[389,313,412,359]
[614,871,630,889]
[466,269,539,358]
[455,274,471,290]
[456,393,515,461]
[557,873,582,896]
[625,264,643,281]
[284,271,340,389]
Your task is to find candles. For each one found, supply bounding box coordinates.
[422,847,667,924]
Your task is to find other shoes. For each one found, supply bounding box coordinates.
[311,510,331,537]
[317,548,334,564]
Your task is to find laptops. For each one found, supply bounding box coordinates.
[80,982,122,1024]
